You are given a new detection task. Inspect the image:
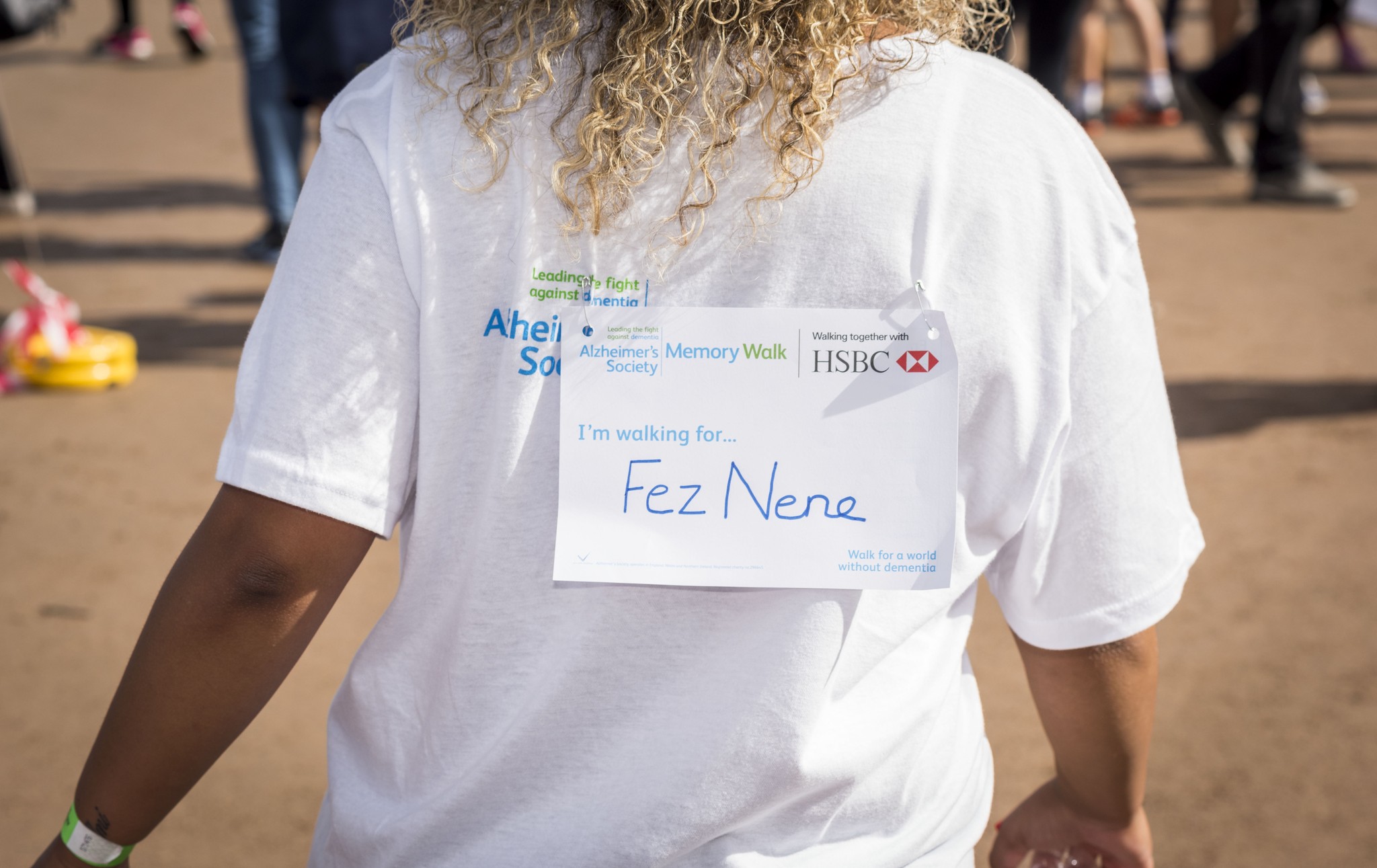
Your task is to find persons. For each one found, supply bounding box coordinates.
[216,0,423,264]
[2,0,1211,868]
[966,0,1377,217]
[85,0,213,61]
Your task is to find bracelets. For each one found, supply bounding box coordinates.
[59,803,136,868]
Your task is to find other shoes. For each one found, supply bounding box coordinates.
[1104,96,1179,128]
[88,26,150,62]
[246,223,288,264]
[1067,89,1106,137]
[1245,162,1360,208]
[174,5,215,60]
[1175,84,1252,171]
